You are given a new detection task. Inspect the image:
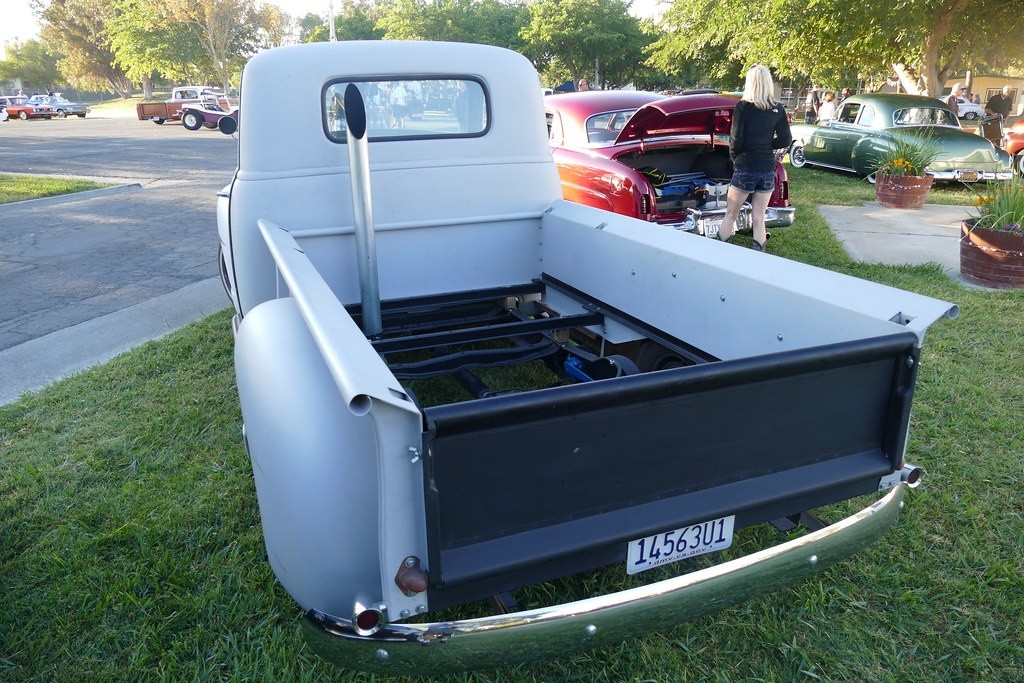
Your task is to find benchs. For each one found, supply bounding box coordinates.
[205,104,224,112]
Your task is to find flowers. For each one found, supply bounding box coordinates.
[949,160,1024,240]
[860,120,948,183]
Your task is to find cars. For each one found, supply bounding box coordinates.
[789,91,1016,188]
[0,96,59,120]
[539,86,795,234]
[30,94,88,118]
[1000,117,1024,178]
[937,95,983,121]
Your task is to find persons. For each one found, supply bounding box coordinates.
[937,83,967,126]
[46,89,55,96]
[804,84,850,125]
[578,78,590,91]
[16,90,28,104]
[968,93,980,104]
[363,81,405,129]
[716,65,792,252]
[985,85,1013,124]
[457,80,483,132]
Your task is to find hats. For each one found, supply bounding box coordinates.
[952,83,967,91]
[810,85,820,92]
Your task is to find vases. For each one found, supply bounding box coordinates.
[875,172,934,210]
[960,218,1024,288]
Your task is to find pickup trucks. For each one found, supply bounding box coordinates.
[136,85,220,125]
[213,40,961,672]
